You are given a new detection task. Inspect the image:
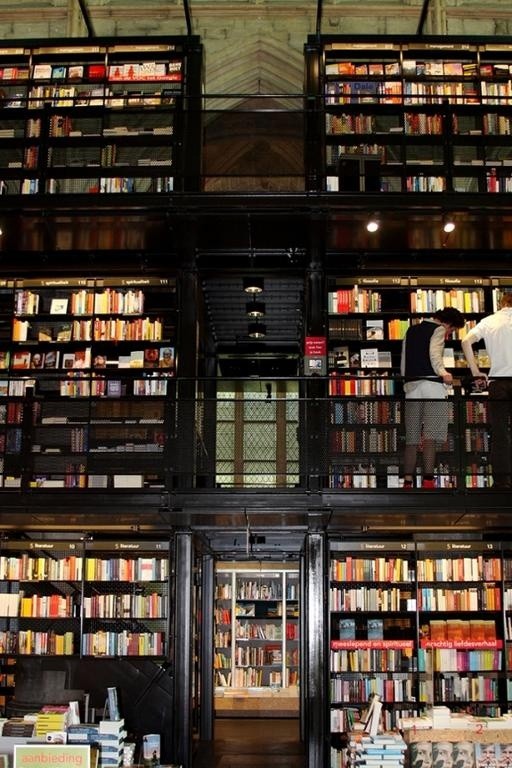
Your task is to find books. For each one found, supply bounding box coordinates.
[215,574,298,691]
[0,550,170,767]
[328,269,511,487]
[331,549,512,768]
[2,52,177,195]
[0,289,180,490]
[322,48,511,191]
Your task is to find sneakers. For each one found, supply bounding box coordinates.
[423,479,437,488]
[404,484,411,490]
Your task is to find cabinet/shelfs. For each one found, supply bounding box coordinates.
[0,281,207,488]
[1,34,206,195]
[302,269,511,488]
[303,31,509,195]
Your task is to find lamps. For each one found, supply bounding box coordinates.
[244,277,269,340]
